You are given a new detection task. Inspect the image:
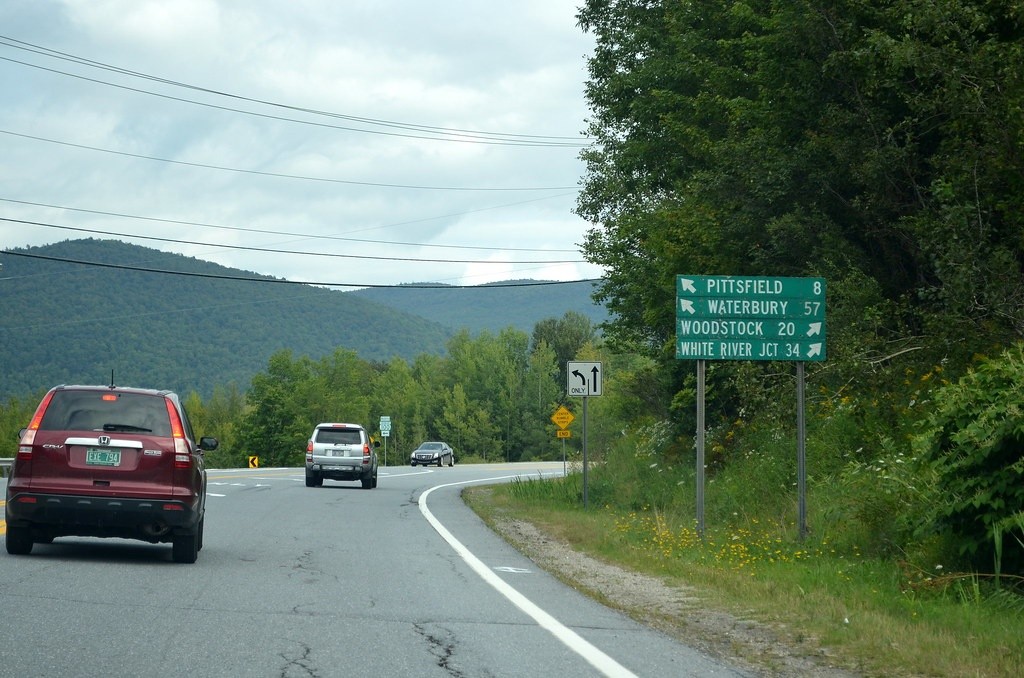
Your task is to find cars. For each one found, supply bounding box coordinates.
[411,442,455,467]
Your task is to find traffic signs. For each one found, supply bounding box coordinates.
[675,273,827,360]
[566,361,602,397]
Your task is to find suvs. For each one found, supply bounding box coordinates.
[6,385,218,564]
[304,422,381,489]
